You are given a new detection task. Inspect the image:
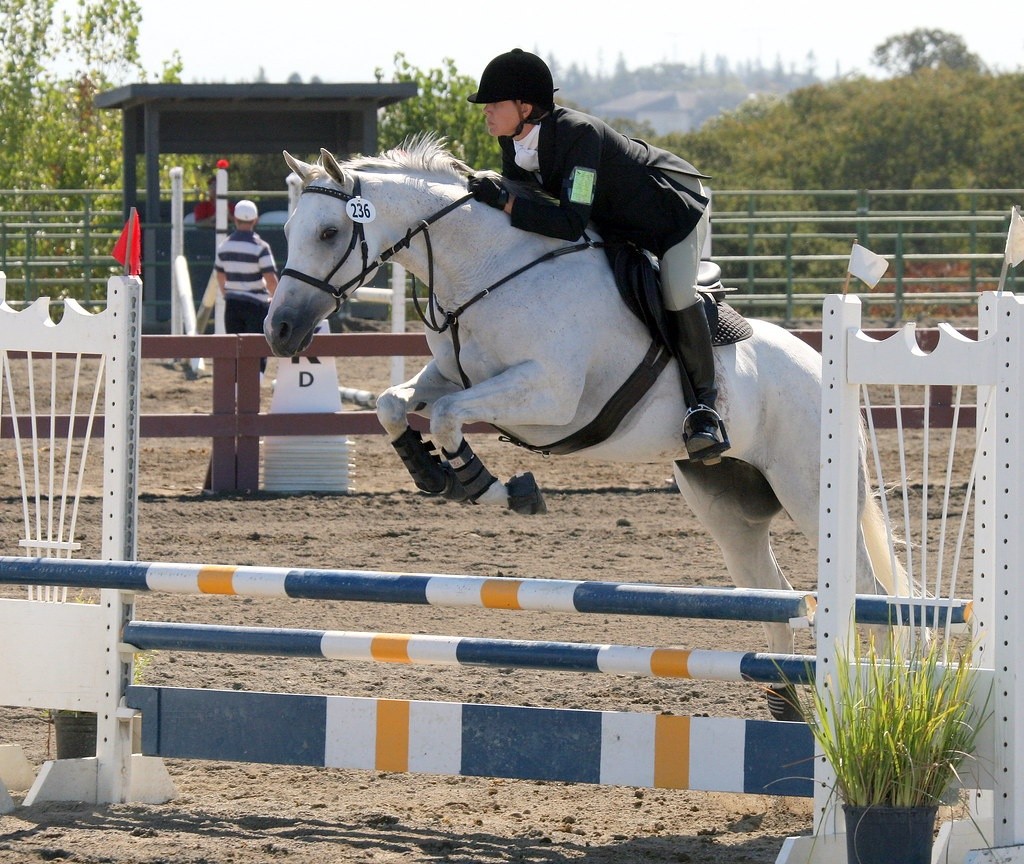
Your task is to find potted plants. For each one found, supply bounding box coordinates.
[760,604,999,864]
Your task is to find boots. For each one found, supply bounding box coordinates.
[663,299,722,468]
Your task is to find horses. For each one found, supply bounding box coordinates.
[264,128,939,722]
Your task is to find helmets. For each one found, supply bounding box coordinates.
[467,48,559,111]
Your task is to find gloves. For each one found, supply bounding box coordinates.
[467,175,510,211]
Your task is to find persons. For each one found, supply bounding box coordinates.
[194,176,280,400]
[467,49,724,465]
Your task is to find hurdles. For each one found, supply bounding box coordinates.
[0,273,1024,864]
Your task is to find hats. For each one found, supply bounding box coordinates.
[233,199,258,221]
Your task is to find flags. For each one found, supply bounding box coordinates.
[1004,206,1024,268]
[847,244,890,290]
[112,206,143,275]
